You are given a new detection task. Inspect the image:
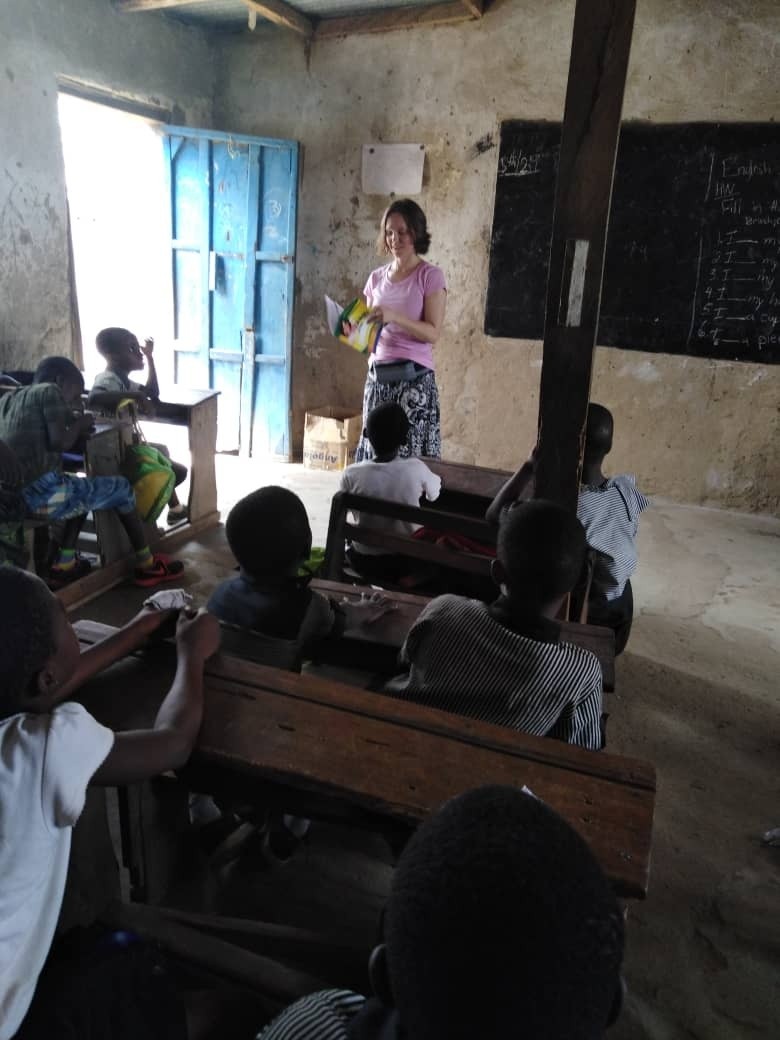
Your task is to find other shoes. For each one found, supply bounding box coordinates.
[260,811,313,863]
[166,505,189,523]
[199,811,257,854]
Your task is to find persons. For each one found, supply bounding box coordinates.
[186,485,390,858]
[353,200,446,464]
[376,501,605,752]
[486,404,651,656]
[338,403,441,586]
[341,319,358,344]
[0,356,184,587]
[0,561,223,1040]
[87,328,188,524]
[257,786,626,1039]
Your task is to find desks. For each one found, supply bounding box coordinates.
[413,457,515,514]
[60,377,223,567]
[72,621,655,957]
[307,577,617,693]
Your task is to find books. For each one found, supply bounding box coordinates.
[325,294,381,353]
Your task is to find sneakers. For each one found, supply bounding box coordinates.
[48,559,94,585]
[137,554,186,587]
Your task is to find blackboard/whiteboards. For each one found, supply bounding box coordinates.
[485,116,780,365]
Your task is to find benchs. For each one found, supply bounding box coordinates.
[317,492,594,618]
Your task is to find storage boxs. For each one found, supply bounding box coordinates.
[303,403,364,471]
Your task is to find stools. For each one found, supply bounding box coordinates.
[17,516,48,577]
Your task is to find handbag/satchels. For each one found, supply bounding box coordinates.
[369,361,431,382]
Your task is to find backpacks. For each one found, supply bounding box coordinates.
[113,399,177,525]
